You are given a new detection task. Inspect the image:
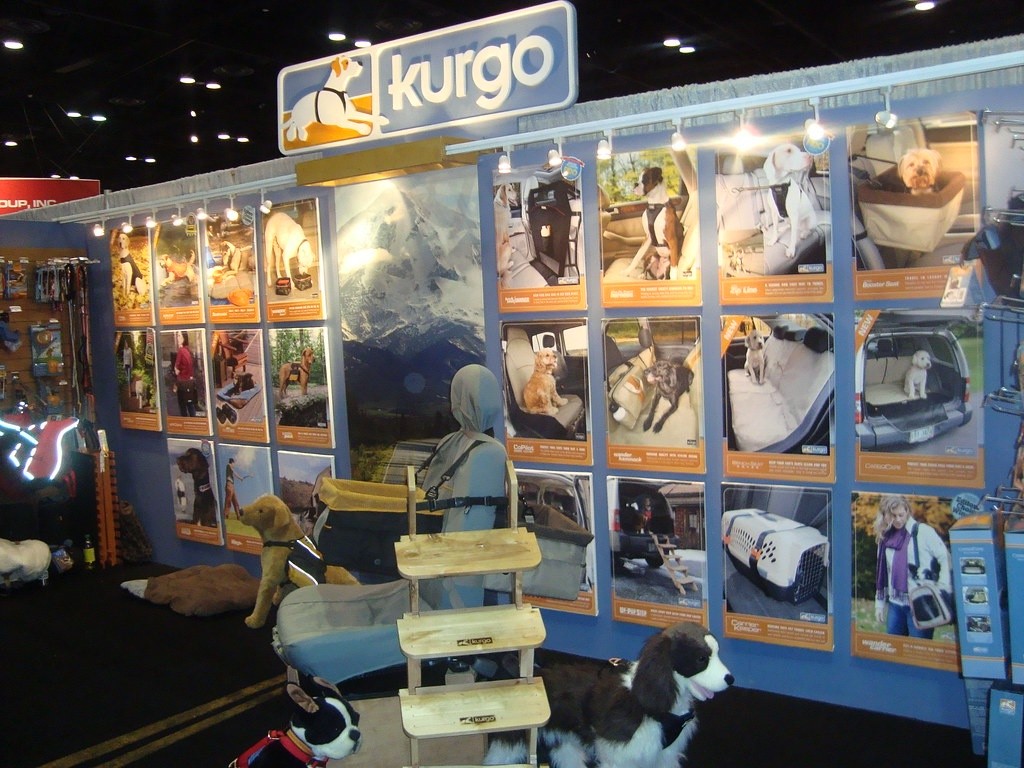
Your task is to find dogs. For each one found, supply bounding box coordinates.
[903,350,931,399]
[264,212,314,289]
[635,497,653,535]
[744,331,765,384]
[279,348,316,398]
[629,168,684,278]
[160,250,195,286]
[898,149,943,194]
[175,448,217,529]
[495,183,517,287]
[232,371,253,393]
[523,351,568,414]
[763,143,830,257]
[643,360,694,433]
[217,241,253,277]
[117,233,145,298]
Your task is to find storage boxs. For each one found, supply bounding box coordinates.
[311,480,447,571]
[4,264,76,420]
[1001,518,1024,685]
[963,678,994,755]
[483,499,594,602]
[985,683,1024,768]
[857,165,966,253]
[949,512,1011,681]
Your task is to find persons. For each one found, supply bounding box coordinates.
[223,458,243,521]
[873,493,952,640]
[174,331,197,417]
[123,338,133,397]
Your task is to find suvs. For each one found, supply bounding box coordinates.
[855,310,974,450]
[606,476,692,569]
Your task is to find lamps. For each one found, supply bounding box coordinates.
[670,118,688,151]
[259,188,272,214]
[121,212,135,234]
[224,194,238,222]
[875,86,898,128]
[93,217,105,237]
[498,144,514,174]
[171,204,185,227]
[805,96,825,141]
[196,199,210,220]
[548,137,567,167]
[596,129,613,160]
[145,207,158,228]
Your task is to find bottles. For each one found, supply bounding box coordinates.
[82,535,96,570]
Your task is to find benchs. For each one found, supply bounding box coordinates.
[865,356,921,415]
[610,342,700,448]
[714,167,830,277]
[724,485,831,623]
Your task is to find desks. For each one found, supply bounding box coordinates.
[0,560,305,768]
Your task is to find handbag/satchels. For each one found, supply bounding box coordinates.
[908,584,951,629]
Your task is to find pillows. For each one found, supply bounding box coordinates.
[144,563,260,619]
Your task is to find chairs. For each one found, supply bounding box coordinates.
[509,181,585,287]
[505,327,585,440]
[601,147,699,282]
[727,310,836,454]
[602,335,621,381]
[273,364,509,687]
[848,165,977,270]
[863,125,980,233]
[509,176,540,273]
[216,332,250,379]
